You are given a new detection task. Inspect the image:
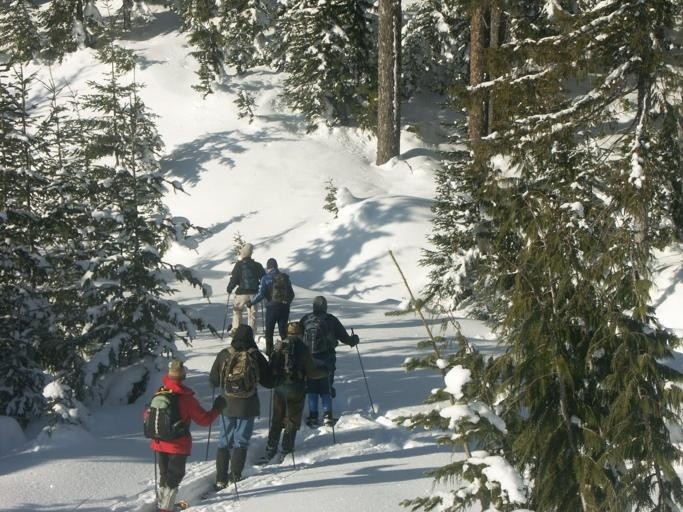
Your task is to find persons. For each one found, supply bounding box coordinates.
[143,360,226,512]
[226,244,266,341]
[246,258,294,356]
[300,296,359,427]
[265,322,330,464]
[210,323,273,488]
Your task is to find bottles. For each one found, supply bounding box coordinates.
[239,244,252,255]
[167,361,185,381]
[288,322,304,335]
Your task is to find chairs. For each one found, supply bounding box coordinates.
[228,473,240,480]
[322,413,335,425]
[266,446,278,458]
[214,481,227,491]
[308,414,317,425]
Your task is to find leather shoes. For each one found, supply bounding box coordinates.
[211,395,228,412]
[224,287,230,294]
[350,335,358,347]
[245,300,251,307]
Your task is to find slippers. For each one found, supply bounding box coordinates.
[306,312,333,367]
[221,346,257,398]
[143,387,187,440]
[241,266,258,290]
[273,274,294,302]
[276,345,304,382]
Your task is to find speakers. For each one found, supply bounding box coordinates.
[156,485,186,512]
